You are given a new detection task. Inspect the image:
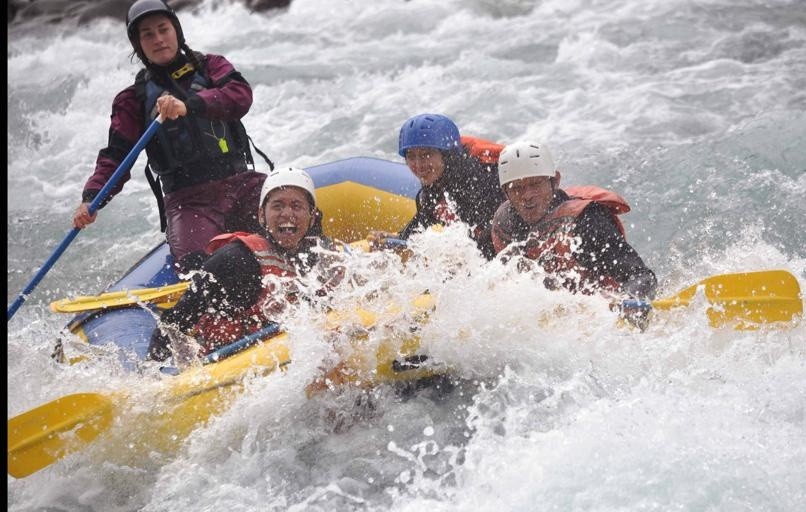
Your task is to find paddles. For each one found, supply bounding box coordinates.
[5,322,282,482]
[49,283,191,315]
[617,269,803,332]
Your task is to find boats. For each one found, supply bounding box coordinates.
[48,157,641,487]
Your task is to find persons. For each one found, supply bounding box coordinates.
[148,166,348,371]
[364,114,506,264]
[473,140,657,335]
[72,1,269,277]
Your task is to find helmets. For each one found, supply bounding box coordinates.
[259,168,316,209]
[126,0,176,38]
[398,113,462,152]
[498,140,556,186]
